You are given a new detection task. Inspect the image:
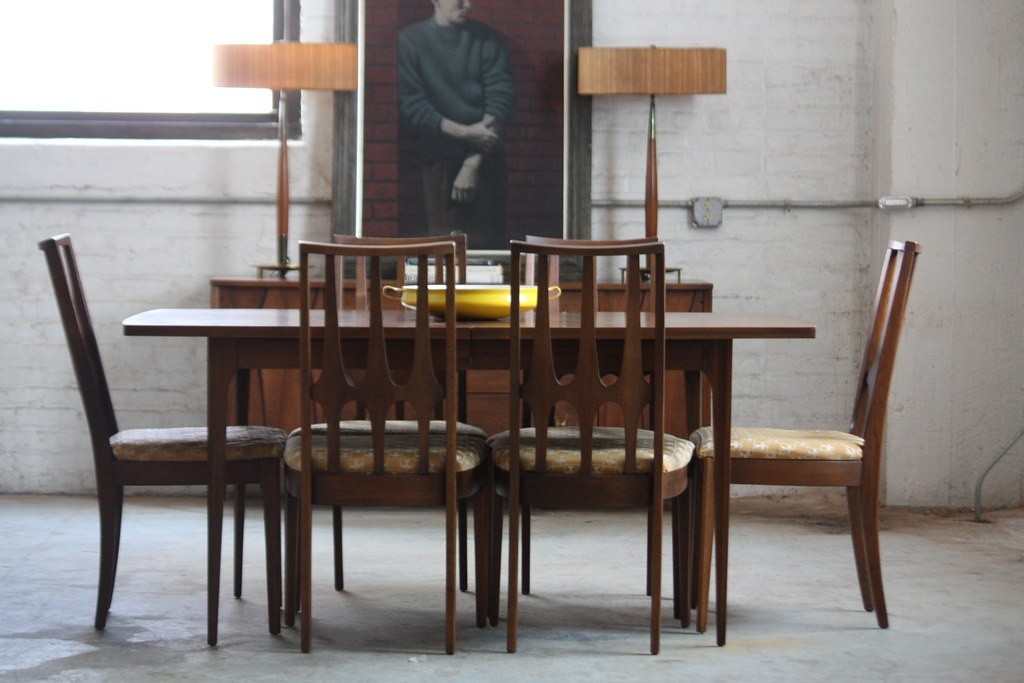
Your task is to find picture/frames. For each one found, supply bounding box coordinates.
[331,0,593,288]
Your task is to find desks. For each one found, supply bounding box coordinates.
[124,307,815,647]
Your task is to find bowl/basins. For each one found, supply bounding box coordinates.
[383,284,561,318]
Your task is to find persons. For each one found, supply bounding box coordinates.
[396,0,514,251]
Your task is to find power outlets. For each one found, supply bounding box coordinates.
[689,195,726,230]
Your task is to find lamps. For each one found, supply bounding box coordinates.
[575,45,730,284]
[216,41,359,279]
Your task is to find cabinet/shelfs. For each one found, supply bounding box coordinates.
[210,279,714,515]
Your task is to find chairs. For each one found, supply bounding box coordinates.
[487,240,696,660]
[519,234,660,598]
[280,238,488,656]
[334,231,470,597]
[38,234,288,636]
[693,240,925,635]
